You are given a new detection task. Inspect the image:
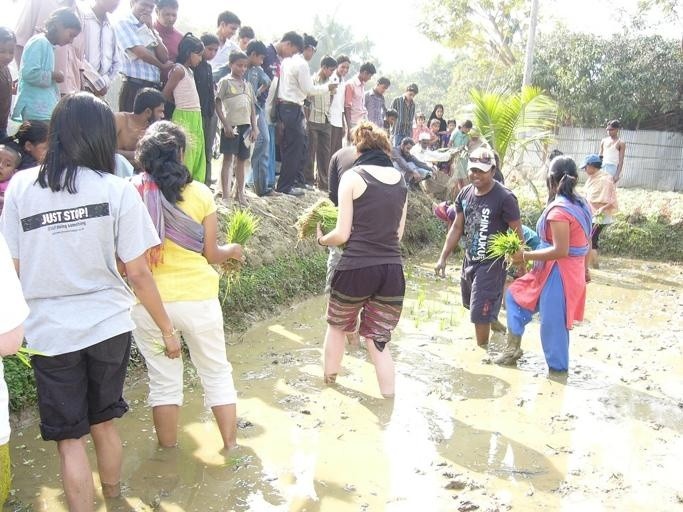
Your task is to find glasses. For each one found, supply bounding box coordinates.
[469,152,494,164]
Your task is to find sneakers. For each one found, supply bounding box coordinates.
[245,182,331,197]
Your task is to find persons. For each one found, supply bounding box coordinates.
[598,119,626,185]
[546,149,564,204]
[579,154,617,282]
[2,0,491,204]
[315,119,409,399]
[434,147,527,347]
[496,155,593,369]
[2,89,247,511]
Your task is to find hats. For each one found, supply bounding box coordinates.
[468,148,497,173]
[580,153,602,170]
